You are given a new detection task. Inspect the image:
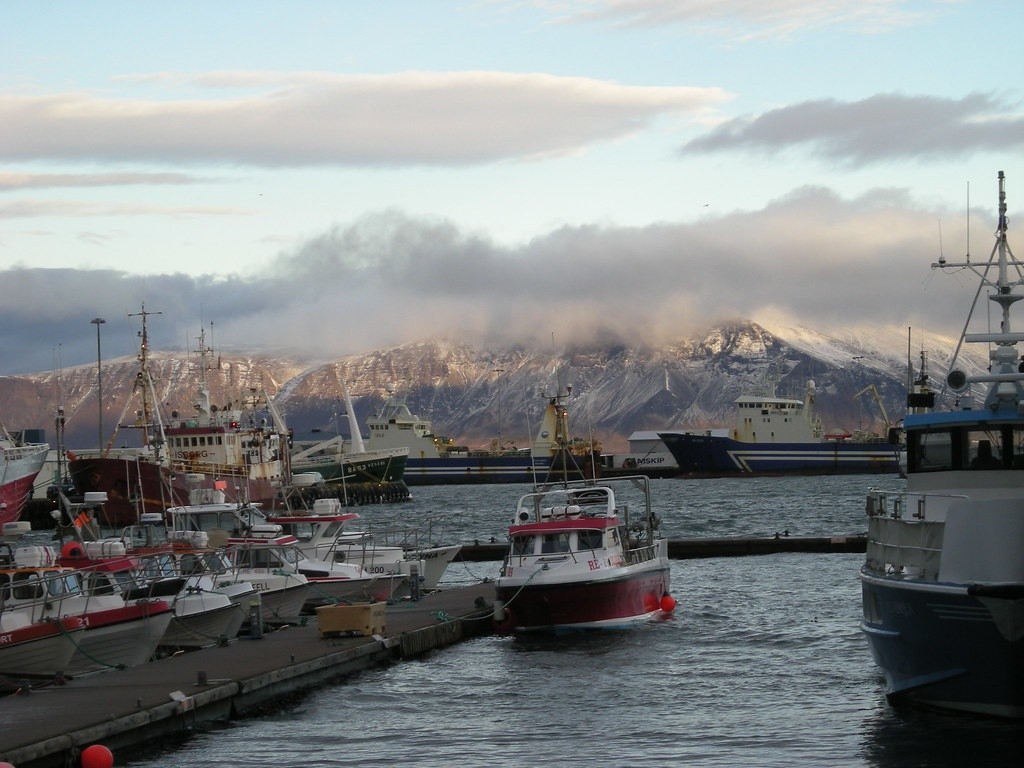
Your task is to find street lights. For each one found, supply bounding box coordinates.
[91,317,109,448]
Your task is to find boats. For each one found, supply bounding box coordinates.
[0,495,468,695]
[0,419,53,538]
[861,169,1024,739]
[654,377,1004,476]
[68,310,292,525]
[287,379,413,509]
[299,332,645,483]
[490,333,678,636]
